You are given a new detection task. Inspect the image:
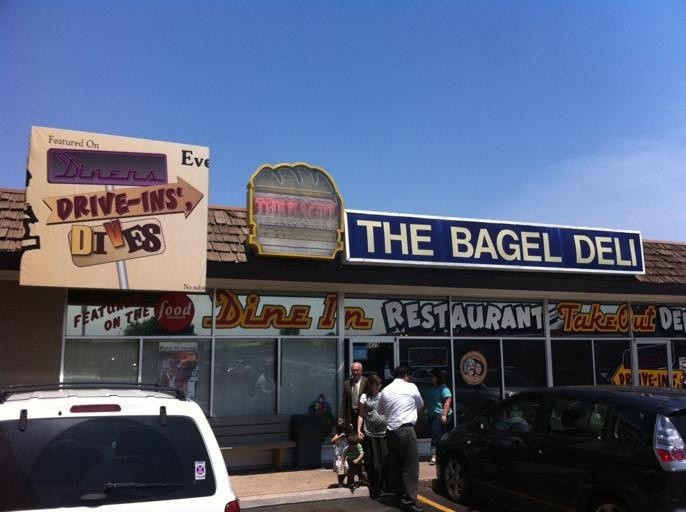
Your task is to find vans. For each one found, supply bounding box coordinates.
[435,384,686,512]
[1,387,240,512]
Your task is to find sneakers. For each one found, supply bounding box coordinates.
[429,457,437,464]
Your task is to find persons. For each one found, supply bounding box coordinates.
[330,362,452,512]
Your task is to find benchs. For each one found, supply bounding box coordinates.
[206,414,296,471]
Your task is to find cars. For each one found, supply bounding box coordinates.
[408,366,532,414]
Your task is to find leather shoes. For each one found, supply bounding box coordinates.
[400,503,424,512]
